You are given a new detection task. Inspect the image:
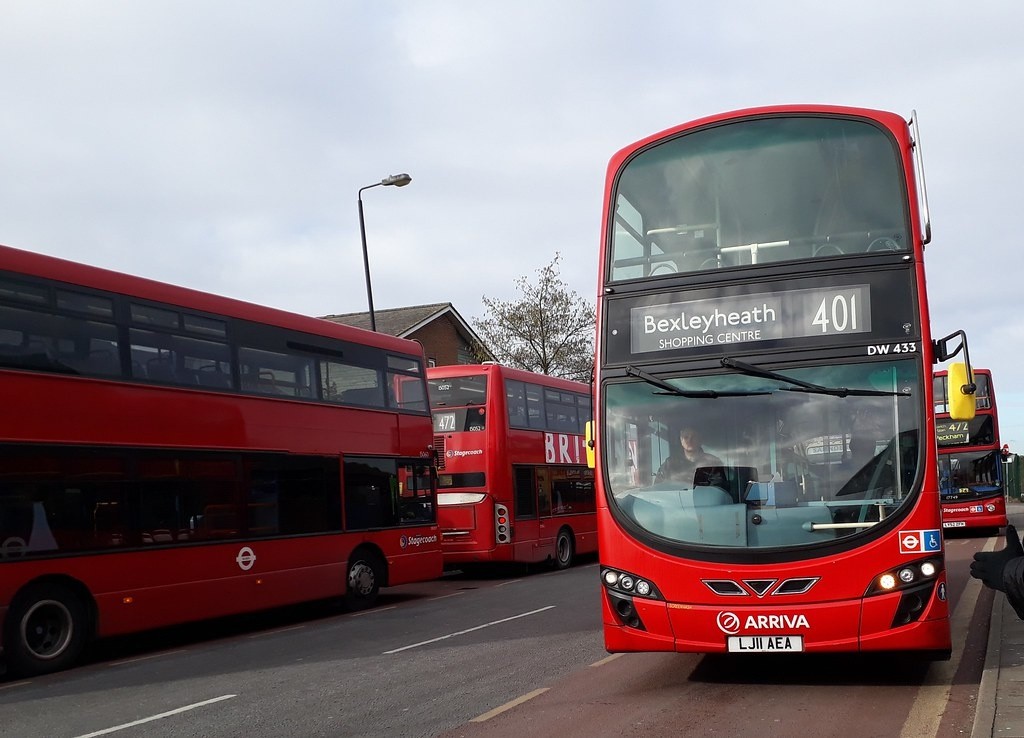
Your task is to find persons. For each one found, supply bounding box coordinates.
[831,432,892,495]
[654,424,724,486]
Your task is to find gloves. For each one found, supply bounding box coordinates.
[970,524,1024,592]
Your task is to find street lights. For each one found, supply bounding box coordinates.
[357,174,413,387]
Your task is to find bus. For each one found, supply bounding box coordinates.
[394,364,641,569]
[589,104,976,654]
[1,244,442,676]
[1,457,279,558]
[933,369,1008,534]
[536,467,595,516]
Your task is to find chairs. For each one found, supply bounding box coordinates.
[18,338,284,387]
[511,409,584,433]
[649,236,902,277]
